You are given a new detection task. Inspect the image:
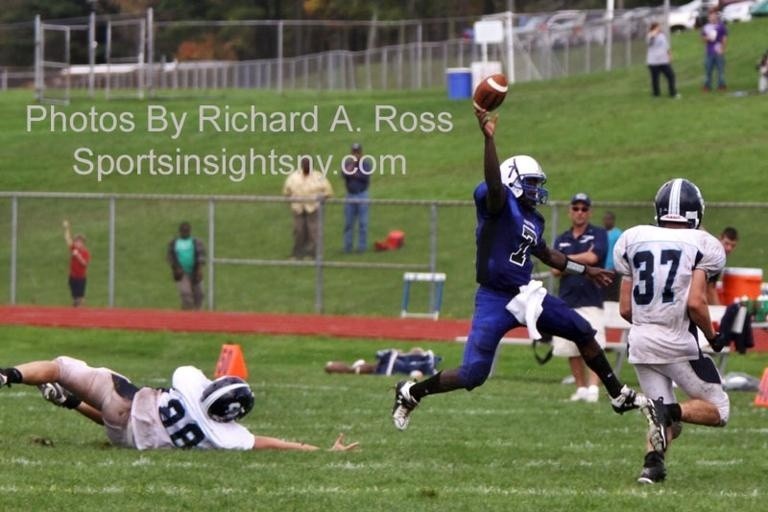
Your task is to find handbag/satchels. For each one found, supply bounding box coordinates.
[373,346,443,379]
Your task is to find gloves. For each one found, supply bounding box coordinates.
[706,330,730,355]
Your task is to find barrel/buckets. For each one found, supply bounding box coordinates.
[722,266,763,307]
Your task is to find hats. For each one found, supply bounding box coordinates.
[569,191,592,207]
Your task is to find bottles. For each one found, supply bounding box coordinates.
[734,294,768,324]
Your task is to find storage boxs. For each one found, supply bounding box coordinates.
[717,267,768,306]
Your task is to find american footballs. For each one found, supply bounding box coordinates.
[352,364,373,375]
[473,74,508,114]
[324,364,351,373]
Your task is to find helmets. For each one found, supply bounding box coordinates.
[653,176,706,229]
[201,373,257,425]
[499,154,548,198]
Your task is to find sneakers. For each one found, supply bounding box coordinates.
[390,379,423,431]
[36,380,64,406]
[636,458,667,487]
[638,394,670,456]
[606,384,651,415]
[673,83,729,98]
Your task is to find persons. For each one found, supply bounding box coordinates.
[282,157,333,259]
[754,49,768,93]
[0,356,360,454]
[699,8,729,92]
[338,142,371,254]
[62,220,90,308]
[646,21,683,99]
[391,106,739,486]
[166,220,206,311]
